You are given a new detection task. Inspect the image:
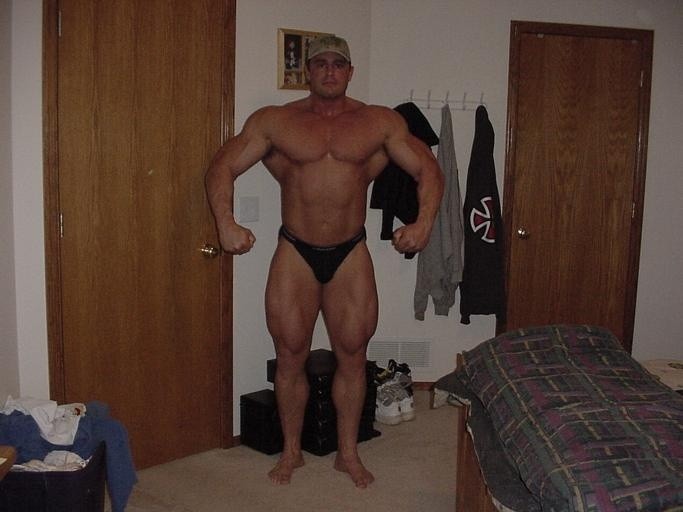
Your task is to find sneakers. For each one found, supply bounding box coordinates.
[371,359,414,397]
[374,393,401,426]
[377,386,416,421]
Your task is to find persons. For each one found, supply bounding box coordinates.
[204,35,445,488]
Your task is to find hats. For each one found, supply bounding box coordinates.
[306,35,351,63]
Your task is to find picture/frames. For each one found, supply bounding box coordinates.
[277,28,334,93]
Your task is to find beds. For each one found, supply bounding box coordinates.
[454,332,683,512]
